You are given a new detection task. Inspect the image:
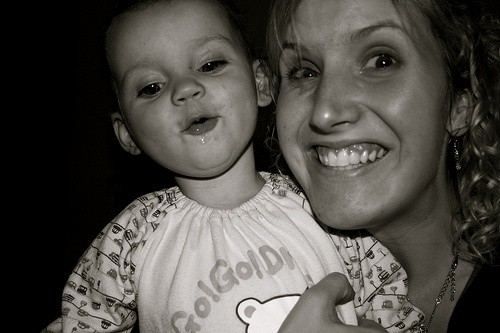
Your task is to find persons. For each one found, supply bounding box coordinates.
[246,0,500,333]
[37,0,430,333]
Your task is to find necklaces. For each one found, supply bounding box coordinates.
[421,248,460,332]
